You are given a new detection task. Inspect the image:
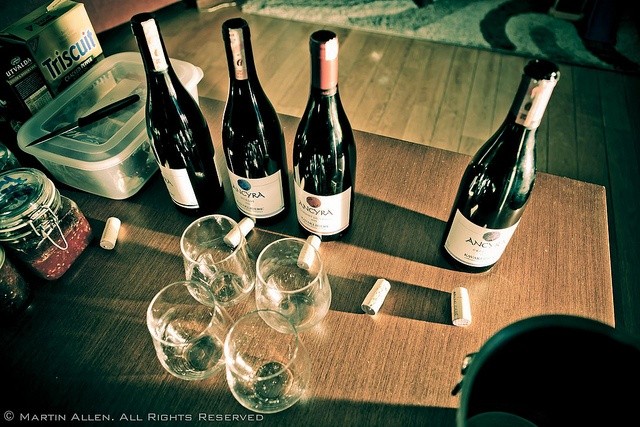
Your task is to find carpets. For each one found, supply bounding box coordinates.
[241,0,608,67]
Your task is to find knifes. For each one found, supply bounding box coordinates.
[24,94,141,149]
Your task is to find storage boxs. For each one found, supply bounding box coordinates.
[16,51,205,201]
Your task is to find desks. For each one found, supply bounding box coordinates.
[0,70,616,425]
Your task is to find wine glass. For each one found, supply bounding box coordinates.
[255,237,332,336]
[146,279,235,381]
[179,214,256,308]
[223,309,311,414]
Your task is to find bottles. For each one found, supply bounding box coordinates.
[1,166,95,283]
[129,12,225,219]
[440,57,560,274]
[293,28,356,242]
[0,246,29,313]
[220,17,290,227]
